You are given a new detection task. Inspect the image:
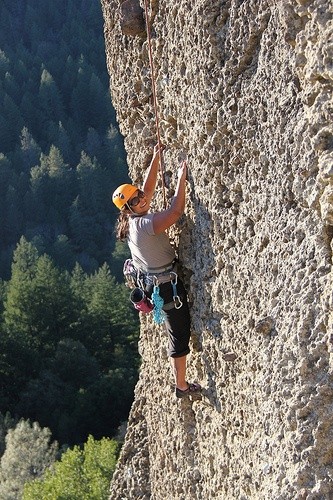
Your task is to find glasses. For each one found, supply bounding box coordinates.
[122,190,145,209]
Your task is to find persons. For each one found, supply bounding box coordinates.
[111,143,200,398]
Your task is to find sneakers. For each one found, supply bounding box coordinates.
[175,382,200,399]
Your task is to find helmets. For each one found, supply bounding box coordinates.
[112,184,140,209]
